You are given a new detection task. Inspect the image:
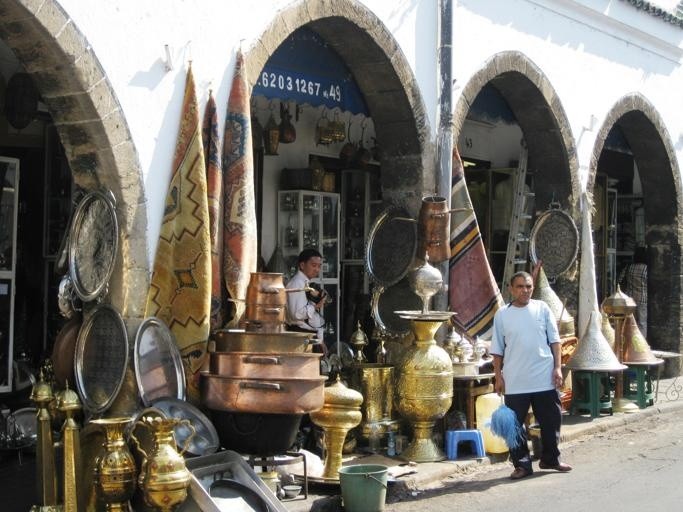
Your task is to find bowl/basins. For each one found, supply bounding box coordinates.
[203,410,304,455]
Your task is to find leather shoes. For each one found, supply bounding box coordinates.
[511,467,532,478]
[539,463,572,471]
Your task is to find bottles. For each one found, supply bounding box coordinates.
[368,423,381,454]
[388,428,396,457]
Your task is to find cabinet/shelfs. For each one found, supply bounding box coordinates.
[277,167,382,357]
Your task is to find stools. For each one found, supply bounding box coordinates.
[443,430,485,460]
[570,365,653,418]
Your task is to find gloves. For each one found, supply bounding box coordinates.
[307,283,327,309]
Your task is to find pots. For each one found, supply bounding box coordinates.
[198,271,326,414]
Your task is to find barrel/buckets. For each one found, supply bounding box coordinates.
[475,393,509,455]
[257,472,280,496]
[338,464,389,512]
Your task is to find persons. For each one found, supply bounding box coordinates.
[280,249,331,353]
[490,272,572,481]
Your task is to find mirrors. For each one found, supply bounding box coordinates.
[363,203,419,290]
